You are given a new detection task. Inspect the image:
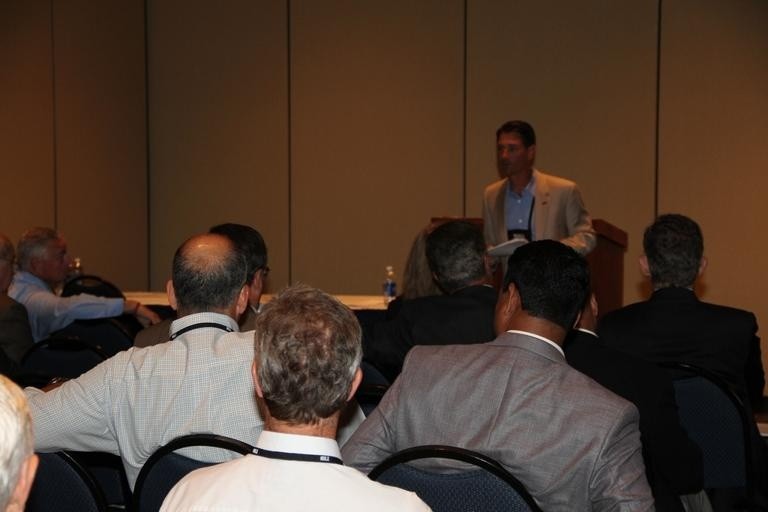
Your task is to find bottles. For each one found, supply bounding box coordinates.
[381,264,397,305]
[73,258,82,274]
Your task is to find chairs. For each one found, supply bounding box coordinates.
[18,343,259,511]
[367,444,539,509]
[608,364,757,511]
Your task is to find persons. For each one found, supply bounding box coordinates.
[20,234,365,492]
[157,283,434,511]
[1,376,41,512]
[342,237,655,511]
[597,213,767,417]
[134,224,269,345]
[480,120,597,330]
[383,218,497,346]
[1,228,160,377]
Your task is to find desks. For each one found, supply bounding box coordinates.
[119,290,394,342]
